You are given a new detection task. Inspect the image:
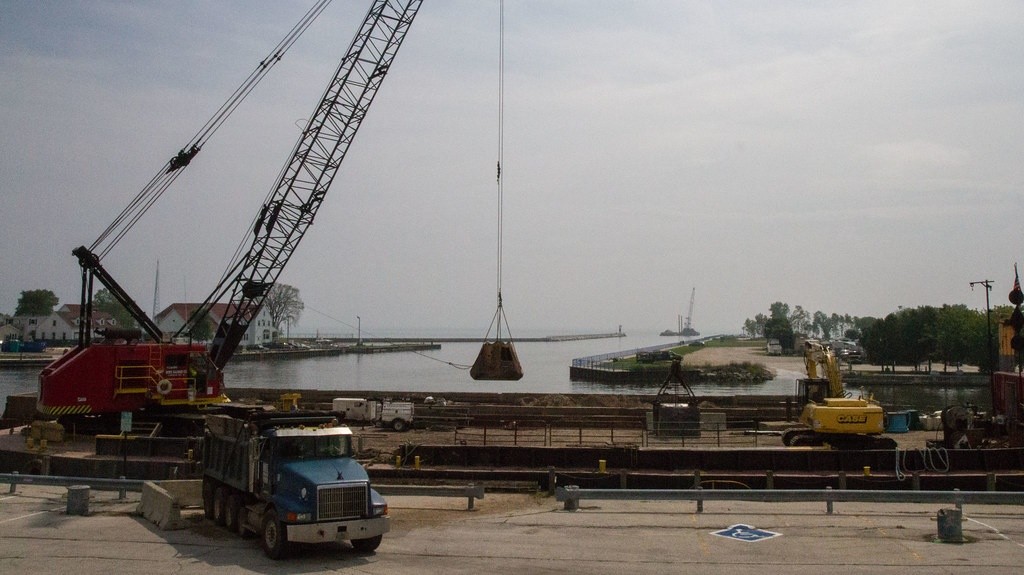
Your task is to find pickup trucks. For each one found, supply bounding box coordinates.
[653,350,683,361]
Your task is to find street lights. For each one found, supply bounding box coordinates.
[356,316,361,347]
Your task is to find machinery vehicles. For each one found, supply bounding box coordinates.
[780,339,898,450]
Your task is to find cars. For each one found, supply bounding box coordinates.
[688,341,705,346]
[637,352,656,364]
[847,350,862,364]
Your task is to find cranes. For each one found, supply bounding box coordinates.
[34,0,425,435]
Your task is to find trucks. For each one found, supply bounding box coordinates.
[330,397,415,433]
[198,406,392,560]
